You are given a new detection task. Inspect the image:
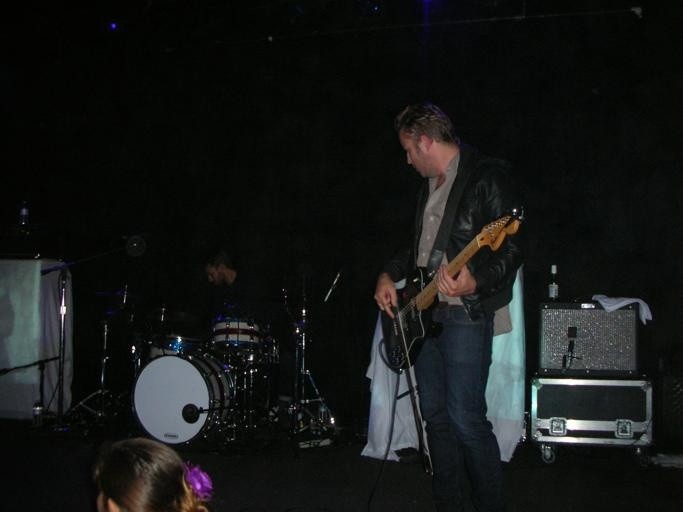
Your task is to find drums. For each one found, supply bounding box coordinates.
[150,334,205,360]
[213,318,264,354]
[134,351,234,447]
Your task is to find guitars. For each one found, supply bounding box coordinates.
[380,202,525,369]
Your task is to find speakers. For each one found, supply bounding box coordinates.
[538,301,638,376]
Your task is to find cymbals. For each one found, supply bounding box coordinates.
[98,289,147,300]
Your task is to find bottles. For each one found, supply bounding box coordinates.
[546,264,560,299]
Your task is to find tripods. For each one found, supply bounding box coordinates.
[68,320,336,442]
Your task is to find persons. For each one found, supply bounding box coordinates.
[372,100,536,512]
[91,437,214,512]
[197,248,270,317]
